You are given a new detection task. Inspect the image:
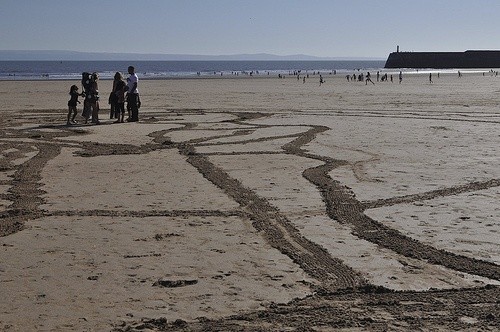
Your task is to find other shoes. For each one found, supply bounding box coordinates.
[66,113,140,124]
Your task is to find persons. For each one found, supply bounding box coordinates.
[126,77,141,120]
[213,70,270,76]
[457,70,462,78]
[80,72,92,97]
[66,85,85,126]
[437,72,441,78]
[126,65,139,122]
[429,73,433,83]
[108,90,118,119]
[41,73,50,78]
[345,71,403,85]
[278,70,336,86]
[482,69,499,76]
[9,74,15,76]
[82,71,100,124]
[112,72,127,123]
[196,71,201,75]
